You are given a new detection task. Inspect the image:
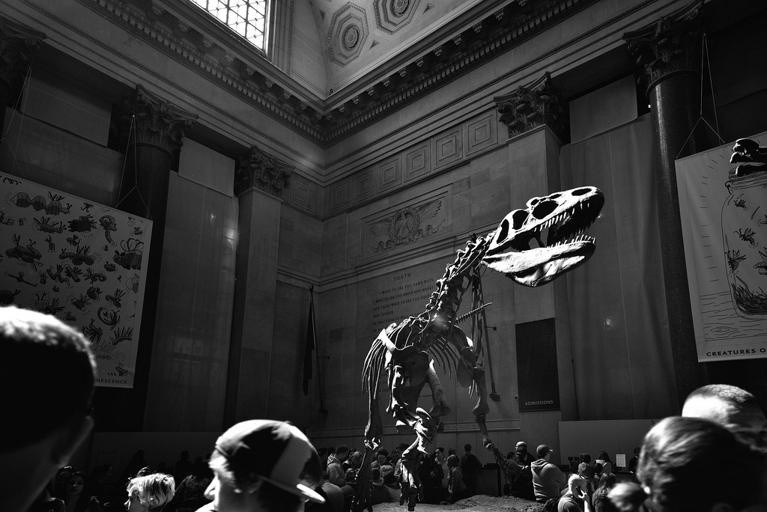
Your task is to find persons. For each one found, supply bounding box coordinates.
[36,465,113,512]
[0,305,97,512]
[420,443,482,505]
[123,472,214,512]
[325,445,400,502]
[192,420,345,512]
[637,384,767,512]
[508,441,641,512]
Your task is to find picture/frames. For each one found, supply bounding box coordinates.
[515,317,560,412]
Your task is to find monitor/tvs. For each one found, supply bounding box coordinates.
[615,454,627,468]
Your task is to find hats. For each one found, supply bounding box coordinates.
[215,419,326,504]
[536,444,553,453]
